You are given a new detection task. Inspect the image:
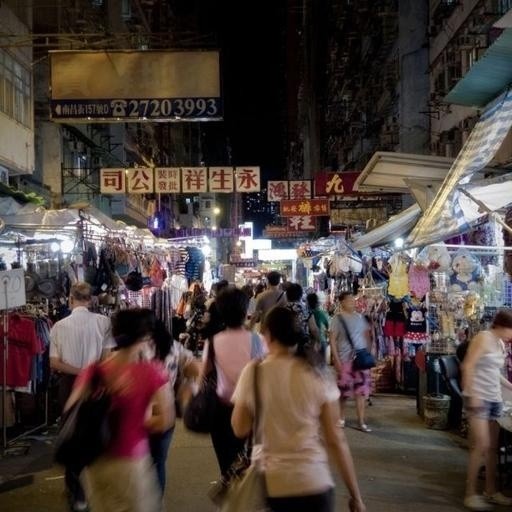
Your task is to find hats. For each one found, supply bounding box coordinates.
[24,271,40,300]
[36,279,56,299]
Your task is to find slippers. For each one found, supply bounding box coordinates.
[336,419,345,429]
[353,425,372,432]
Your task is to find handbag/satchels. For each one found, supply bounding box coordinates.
[52,359,120,466]
[71,239,77,262]
[337,313,375,371]
[76,240,83,264]
[84,246,99,282]
[184,335,223,434]
[207,362,271,512]
[126,252,143,291]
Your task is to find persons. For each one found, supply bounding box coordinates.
[61,308,177,512]
[204,282,225,338]
[254,271,286,320]
[254,282,266,300]
[198,287,271,482]
[329,291,373,433]
[183,283,206,353]
[131,319,208,499]
[280,284,320,355]
[459,310,512,511]
[229,310,367,511]
[241,285,255,310]
[49,282,118,413]
[305,293,329,362]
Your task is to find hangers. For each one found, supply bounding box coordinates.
[10,303,43,318]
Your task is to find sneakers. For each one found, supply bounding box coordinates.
[483,490,512,507]
[72,502,87,512]
[463,494,495,511]
